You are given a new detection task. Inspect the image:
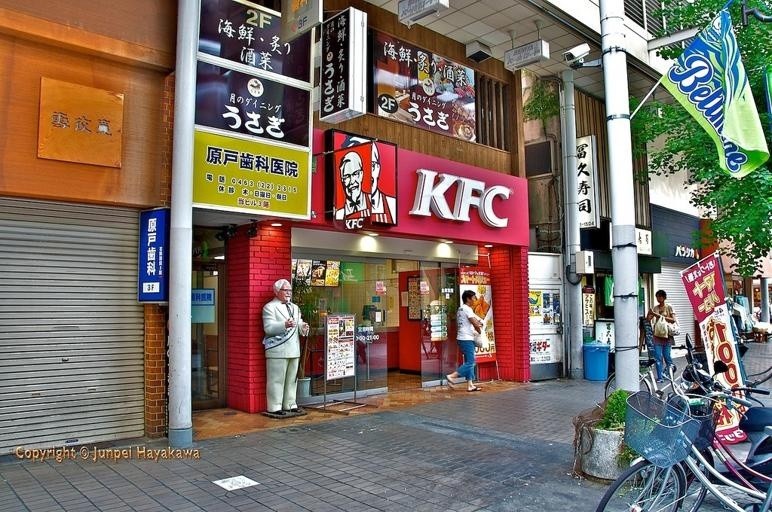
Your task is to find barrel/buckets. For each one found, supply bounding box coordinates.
[584,343,610,382]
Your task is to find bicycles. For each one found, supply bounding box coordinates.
[596,332,772,512]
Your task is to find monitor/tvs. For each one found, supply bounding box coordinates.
[375,310,385,323]
[362,304,377,319]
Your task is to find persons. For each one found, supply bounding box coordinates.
[647,290,677,383]
[263,279,310,415]
[446,290,484,391]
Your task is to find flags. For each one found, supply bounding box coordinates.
[660,5,770,179]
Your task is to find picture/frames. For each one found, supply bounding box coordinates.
[406,275,423,321]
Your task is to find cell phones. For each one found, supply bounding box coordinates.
[649,308,654,313]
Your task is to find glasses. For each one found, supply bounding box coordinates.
[342,169,363,183]
[280,288,292,292]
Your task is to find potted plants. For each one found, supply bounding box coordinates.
[294,260,321,399]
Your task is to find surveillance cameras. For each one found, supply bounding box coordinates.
[563,43,591,64]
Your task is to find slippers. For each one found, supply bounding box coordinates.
[468,387,482,391]
[446,375,457,385]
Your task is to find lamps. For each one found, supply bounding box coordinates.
[464,41,492,64]
